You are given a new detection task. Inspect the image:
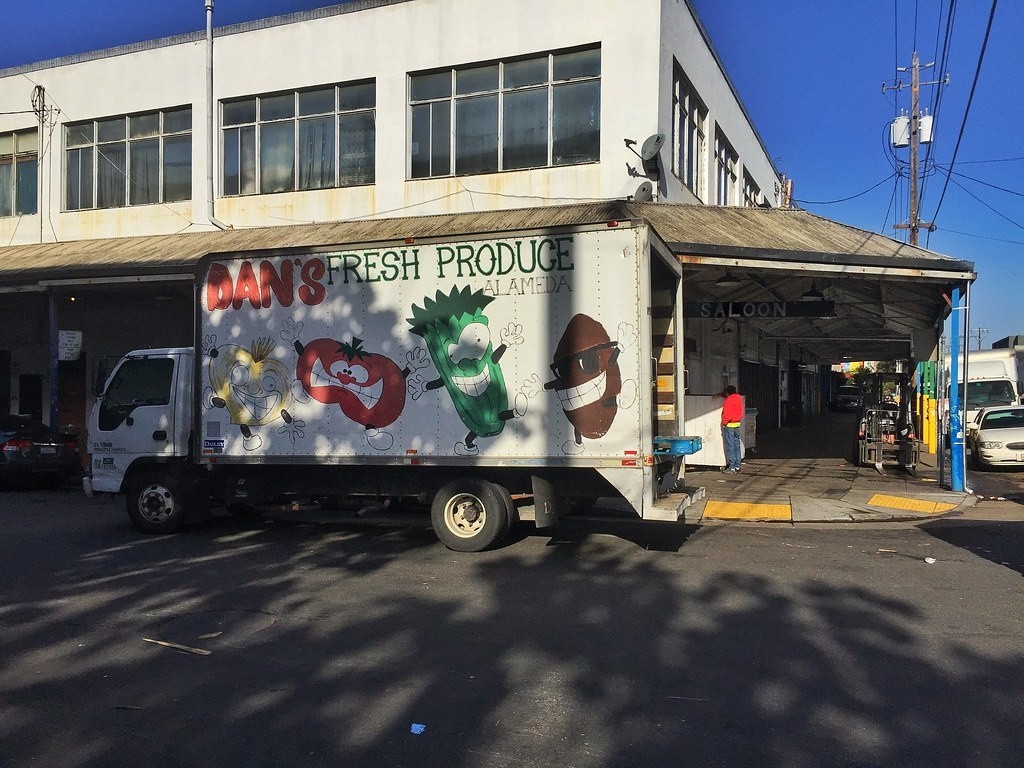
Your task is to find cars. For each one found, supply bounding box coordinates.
[0,414,82,492]
[829,385,863,410]
[966,405,1024,471]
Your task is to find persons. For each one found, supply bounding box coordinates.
[712,385,744,474]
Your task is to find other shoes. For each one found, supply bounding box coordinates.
[722,467,735,474]
[735,467,742,473]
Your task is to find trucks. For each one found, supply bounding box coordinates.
[941,347,1024,451]
[82,218,707,552]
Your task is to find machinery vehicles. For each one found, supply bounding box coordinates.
[857,372,921,478]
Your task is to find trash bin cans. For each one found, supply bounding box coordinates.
[784,400,805,427]
